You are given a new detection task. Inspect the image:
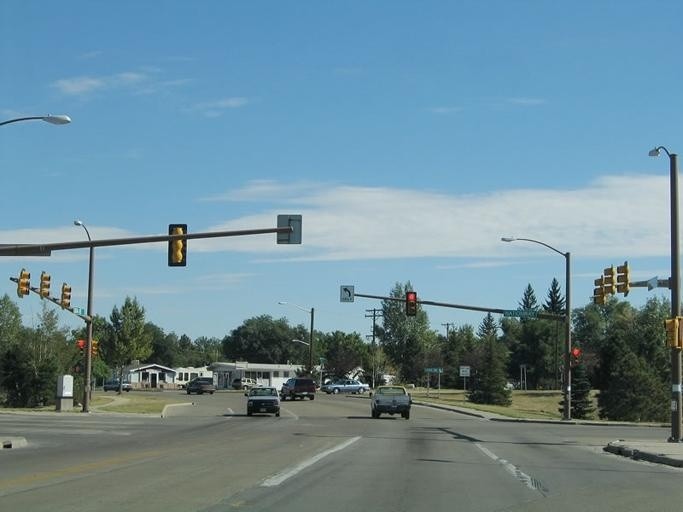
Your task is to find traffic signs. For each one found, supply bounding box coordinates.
[340,285,354,302]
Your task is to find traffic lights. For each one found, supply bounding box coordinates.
[406,292,416,316]
[594,261,630,305]
[40,271,51,300]
[17,268,30,299]
[572,341,583,367]
[76,340,98,358]
[60,284,71,309]
[664,316,683,349]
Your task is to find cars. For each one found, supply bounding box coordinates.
[177,377,216,394]
[232,378,370,416]
[103,378,132,392]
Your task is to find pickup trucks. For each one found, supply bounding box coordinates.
[369,384,412,419]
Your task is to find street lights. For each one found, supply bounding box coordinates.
[648,144,681,443]
[0,116,72,126]
[502,237,572,419]
[73,218,94,413]
[278,301,314,367]
